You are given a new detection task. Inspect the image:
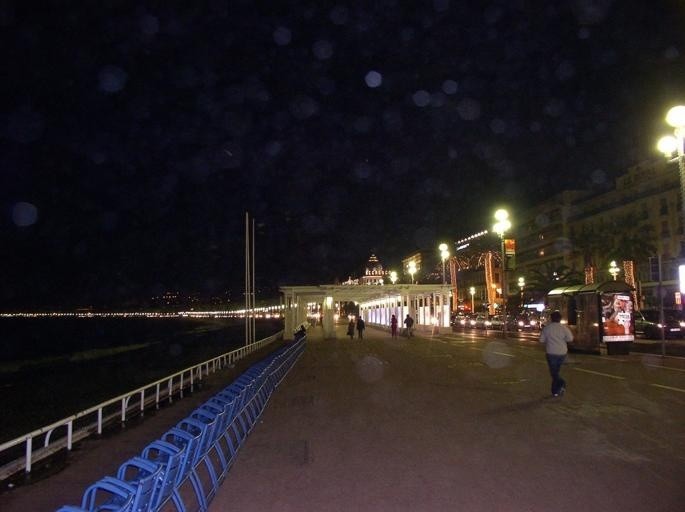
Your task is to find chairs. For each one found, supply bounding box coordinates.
[56,348,297,511]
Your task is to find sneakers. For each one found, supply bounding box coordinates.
[552,382,567,398]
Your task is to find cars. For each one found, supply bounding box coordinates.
[450,302,685,339]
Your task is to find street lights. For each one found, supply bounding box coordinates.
[387,208,513,315]
[657,103,685,223]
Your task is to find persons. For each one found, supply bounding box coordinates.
[605,311,626,335]
[538,311,574,398]
[403,314,414,339]
[356,316,366,341]
[390,314,398,339]
[347,318,356,341]
